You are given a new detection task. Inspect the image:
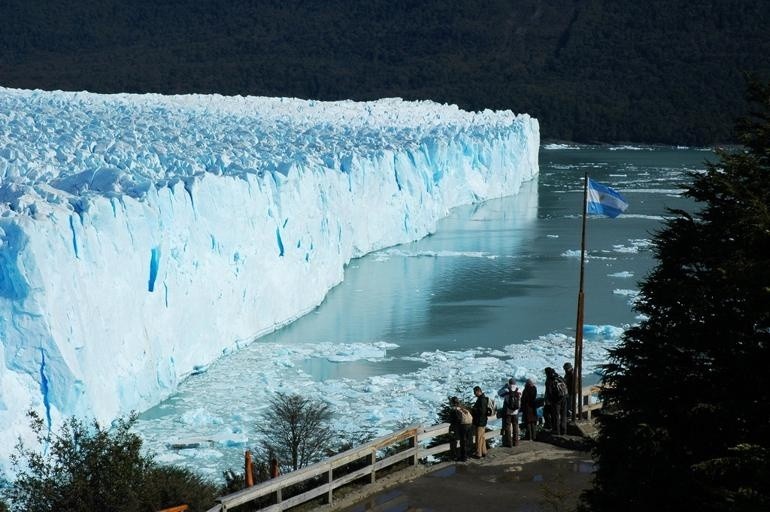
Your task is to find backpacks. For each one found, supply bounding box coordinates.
[547,373,568,398]
[507,387,520,411]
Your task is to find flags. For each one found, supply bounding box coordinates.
[586,178,629,219]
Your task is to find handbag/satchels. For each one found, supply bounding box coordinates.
[461,412,473,424]
[487,397,497,416]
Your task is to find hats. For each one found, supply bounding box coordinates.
[563,363,572,368]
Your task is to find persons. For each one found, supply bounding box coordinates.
[447,396,474,461]
[498,378,522,447]
[563,362,573,416]
[519,379,538,440]
[470,386,488,459]
[545,367,567,435]
